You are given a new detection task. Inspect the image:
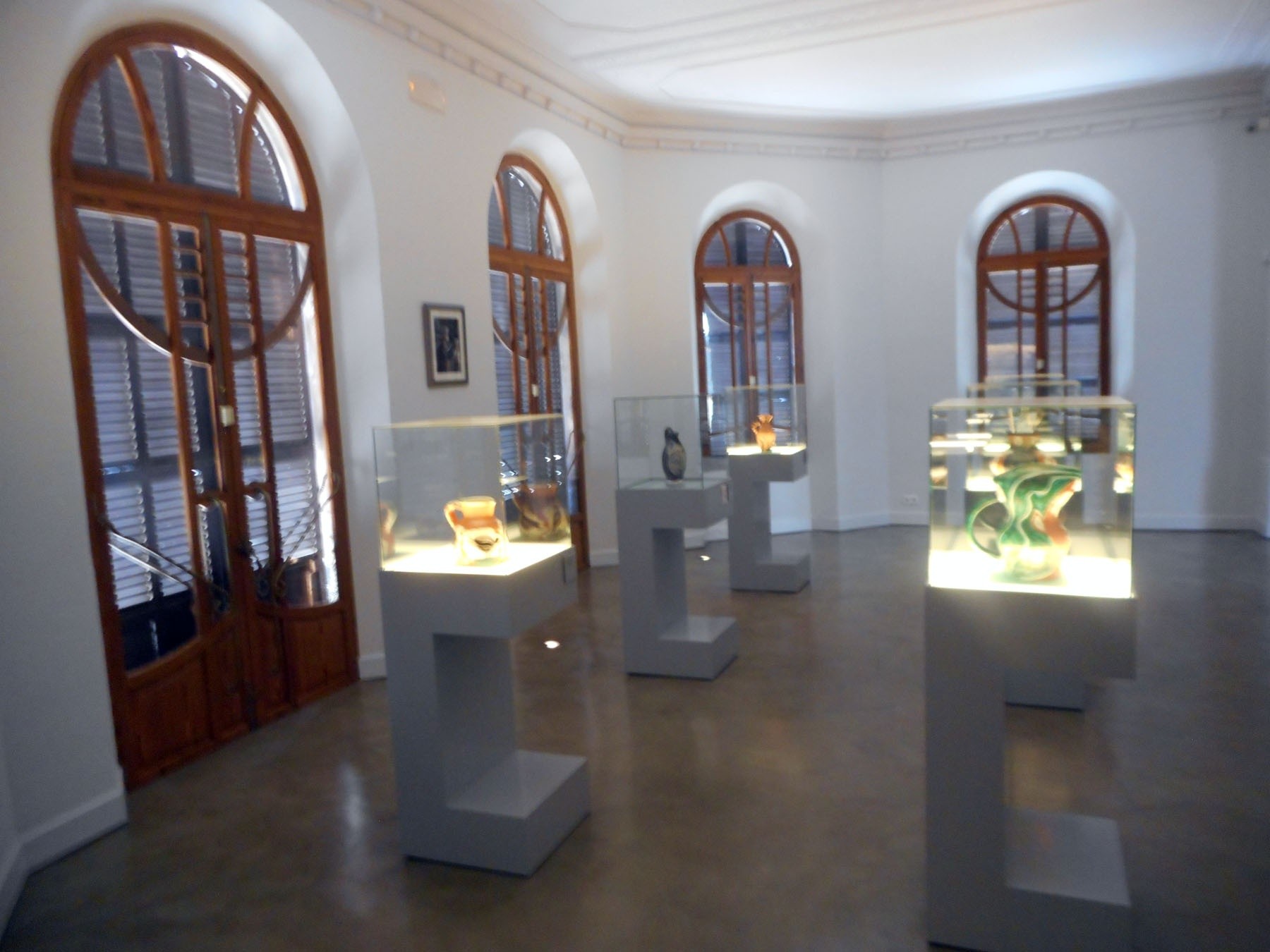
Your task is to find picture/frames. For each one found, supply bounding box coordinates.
[421,301,470,389]
